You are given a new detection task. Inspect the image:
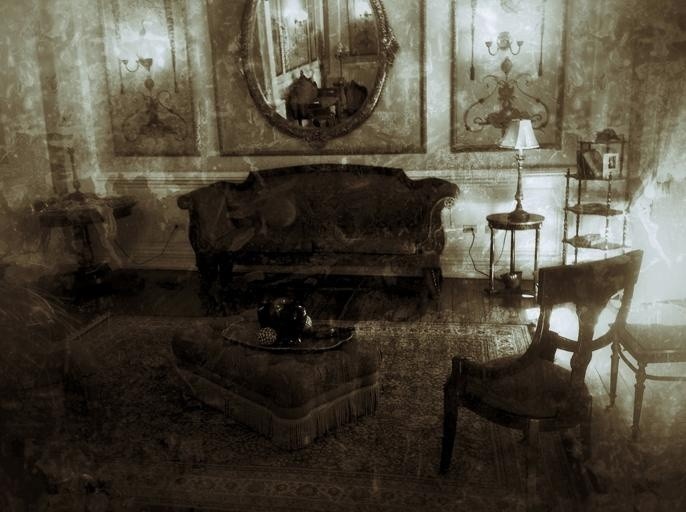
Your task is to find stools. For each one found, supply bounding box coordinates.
[487,213,545,306]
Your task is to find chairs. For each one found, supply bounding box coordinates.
[437,248,643,503]
[609,323,686,441]
[285,69,337,126]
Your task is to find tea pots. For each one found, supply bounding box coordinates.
[258,299,307,345]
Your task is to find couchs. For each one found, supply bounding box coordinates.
[175,163,460,298]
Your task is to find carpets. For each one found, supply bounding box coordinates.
[44,309,590,512]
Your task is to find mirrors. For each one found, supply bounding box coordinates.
[242,0,402,140]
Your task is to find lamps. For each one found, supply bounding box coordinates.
[334,40,348,90]
[498,118,540,222]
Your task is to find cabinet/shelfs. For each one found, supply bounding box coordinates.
[562,127,629,268]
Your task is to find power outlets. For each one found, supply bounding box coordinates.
[173,223,185,233]
[463,225,478,234]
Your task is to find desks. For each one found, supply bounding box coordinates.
[168,309,381,449]
